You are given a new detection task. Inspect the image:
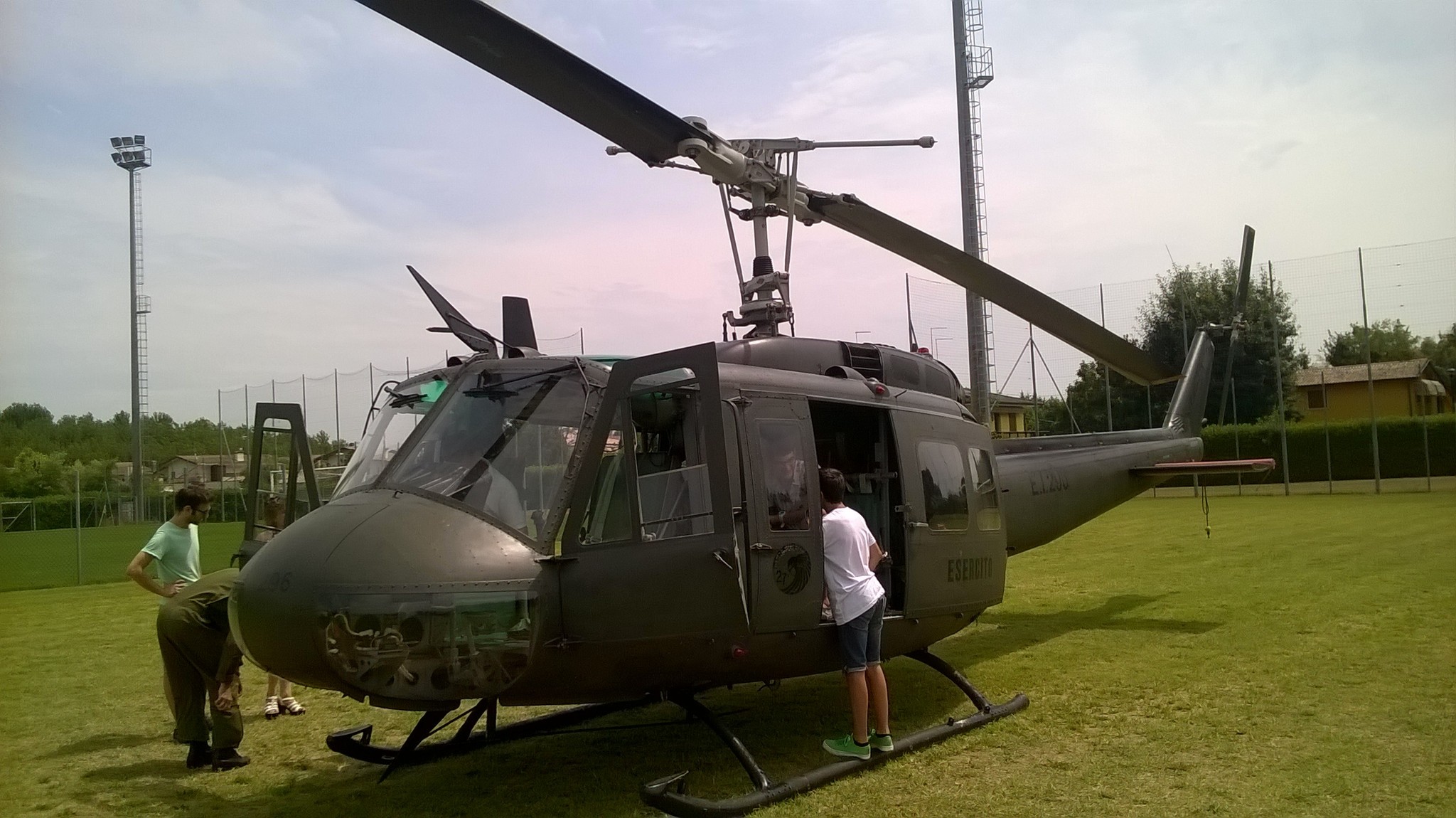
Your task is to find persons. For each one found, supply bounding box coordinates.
[253,496,307,719]
[437,425,530,541]
[157,567,252,771]
[125,484,214,744]
[816,467,894,760]
[764,443,837,623]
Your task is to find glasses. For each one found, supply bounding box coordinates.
[191,506,212,514]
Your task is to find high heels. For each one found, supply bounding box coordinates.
[279,696,305,718]
[264,694,280,720]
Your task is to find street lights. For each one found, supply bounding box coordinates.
[110,134,151,523]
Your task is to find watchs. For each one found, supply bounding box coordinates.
[778,510,786,528]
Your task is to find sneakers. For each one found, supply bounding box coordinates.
[867,728,896,751]
[823,734,871,760]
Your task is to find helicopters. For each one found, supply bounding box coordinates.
[231,0,1276,815]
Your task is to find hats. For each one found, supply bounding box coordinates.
[441,432,477,461]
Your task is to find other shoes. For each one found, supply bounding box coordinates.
[183,739,213,769]
[211,746,251,772]
